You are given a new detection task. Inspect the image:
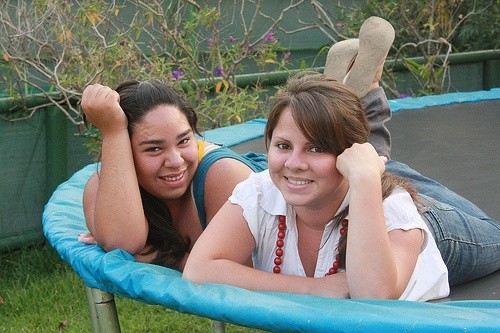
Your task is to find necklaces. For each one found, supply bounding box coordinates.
[273,215,348,277]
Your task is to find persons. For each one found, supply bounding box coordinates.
[182,74,500,300]
[79,16,395,274]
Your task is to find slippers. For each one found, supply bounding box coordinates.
[324,16,396,97]
